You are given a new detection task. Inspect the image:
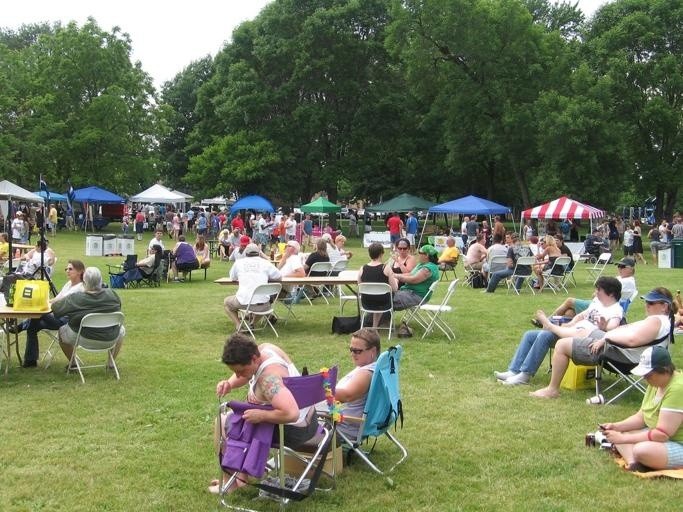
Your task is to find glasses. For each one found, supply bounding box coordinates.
[349,347,372,356]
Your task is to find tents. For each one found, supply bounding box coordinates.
[301,197,341,237]
[363,193,448,229]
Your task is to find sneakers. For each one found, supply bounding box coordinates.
[260,472,312,502]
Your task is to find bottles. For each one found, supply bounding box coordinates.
[15,248,20,259]
[270,248,274,260]
[587,433,596,447]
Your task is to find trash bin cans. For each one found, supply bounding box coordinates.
[657,238,683,269]
[86,233,135,256]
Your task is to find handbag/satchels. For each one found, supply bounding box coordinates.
[2,271,50,311]
[397,321,415,338]
[560,358,595,391]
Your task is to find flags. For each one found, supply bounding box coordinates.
[67,183,75,203]
[39,181,49,195]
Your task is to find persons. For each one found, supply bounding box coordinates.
[122,204,211,283]
[530,257,637,328]
[59,206,84,230]
[492,275,623,385]
[347,208,372,236]
[443,212,682,292]
[386,210,419,254]
[207,331,317,494]
[210,205,353,334]
[358,238,440,327]
[317,326,380,441]
[526,286,675,397]
[599,345,682,471]
[0,202,57,281]
[8,259,125,371]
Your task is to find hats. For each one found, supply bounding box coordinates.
[640,291,673,307]
[630,346,676,377]
[245,243,260,256]
[615,256,636,268]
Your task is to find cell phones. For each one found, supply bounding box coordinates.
[598,423,606,430]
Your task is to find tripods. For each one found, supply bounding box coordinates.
[29,227,58,298]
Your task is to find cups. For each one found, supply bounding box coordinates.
[586,394,605,405]
